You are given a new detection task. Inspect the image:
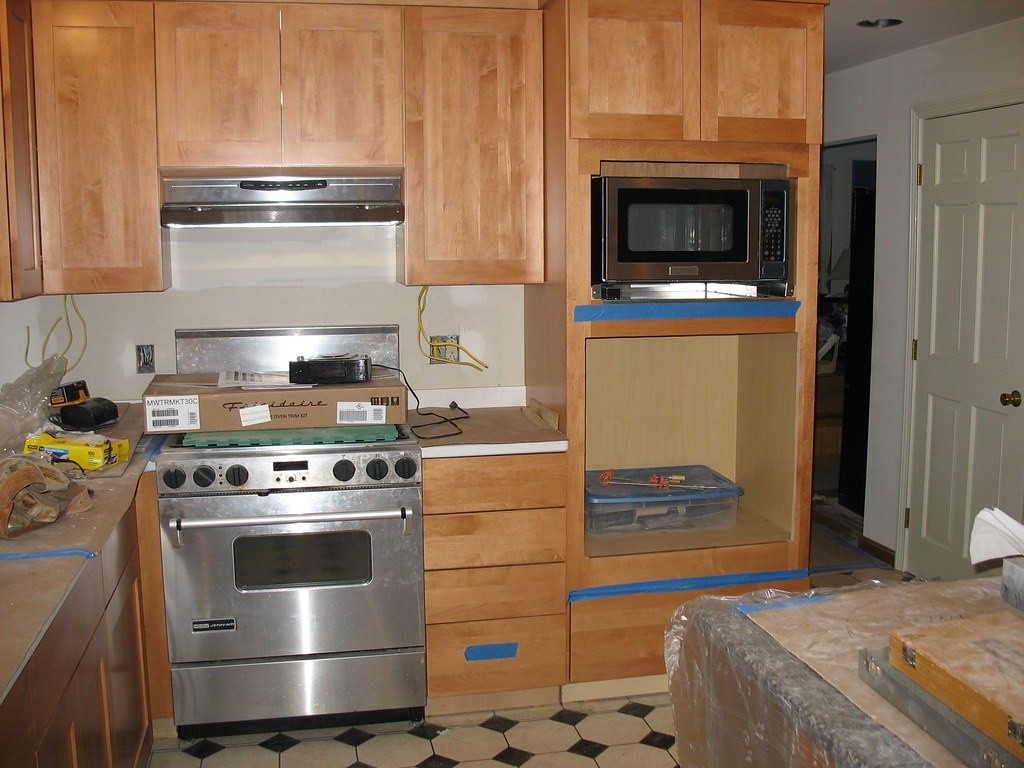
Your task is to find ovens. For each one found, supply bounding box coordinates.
[155,485,425,739]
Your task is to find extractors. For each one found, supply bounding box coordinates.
[162,175,404,228]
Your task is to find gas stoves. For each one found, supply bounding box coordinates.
[155,324,423,493]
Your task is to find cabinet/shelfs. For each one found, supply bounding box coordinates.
[0,401,160,768]
[1,0,545,305]
[403,0,832,718]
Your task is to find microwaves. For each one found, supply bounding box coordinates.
[595,177,791,282]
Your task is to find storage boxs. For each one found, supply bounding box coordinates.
[585,465,745,537]
[49,380,90,407]
[24,432,130,472]
[142,367,408,434]
